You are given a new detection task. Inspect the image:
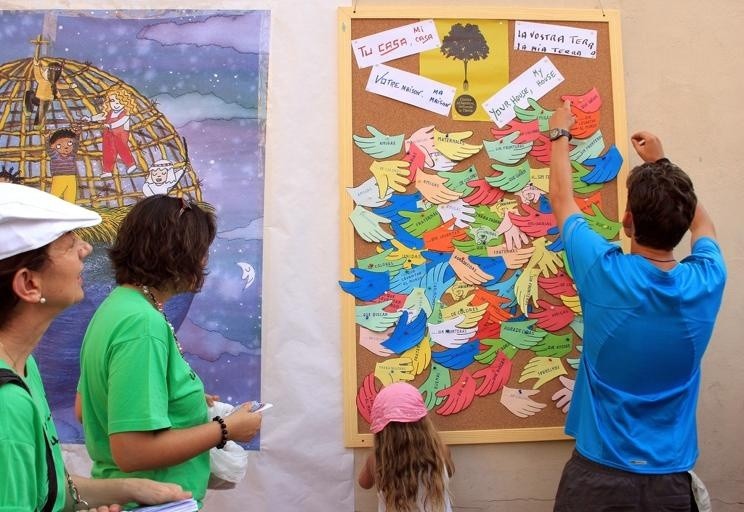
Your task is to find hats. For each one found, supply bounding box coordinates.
[0,181,104,261]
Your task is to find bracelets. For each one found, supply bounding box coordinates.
[213,416,228,449]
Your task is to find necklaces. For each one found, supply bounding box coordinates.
[139,285,186,361]
[642,255,675,263]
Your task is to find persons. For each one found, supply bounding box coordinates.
[358,383,456,512]
[141,159,192,198]
[0,166,25,185]
[39,126,81,204]
[24,58,64,130]
[80,83,137,179]
[547,97,728,511]
[74,194,261,511]
[0,182,196,512]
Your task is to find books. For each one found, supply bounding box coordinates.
[130,498,199,512]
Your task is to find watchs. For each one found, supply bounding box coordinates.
[549,128,572,141]
